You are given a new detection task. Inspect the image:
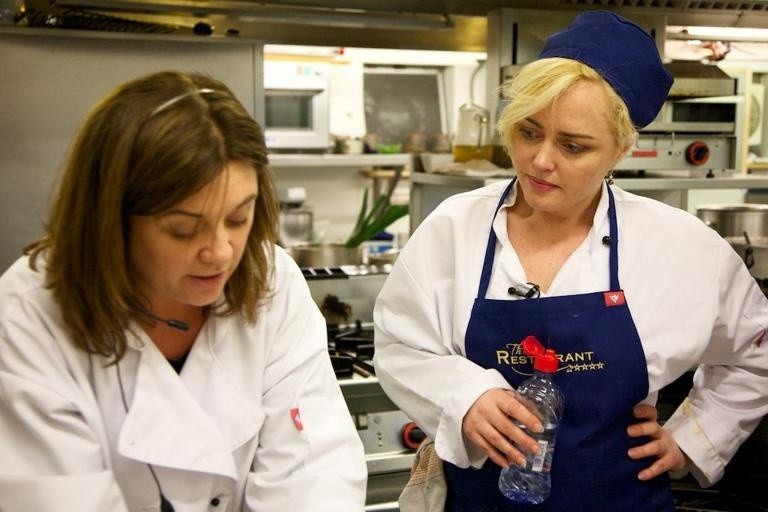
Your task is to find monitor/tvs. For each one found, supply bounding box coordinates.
[364,68,449,153]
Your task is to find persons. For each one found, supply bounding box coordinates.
[369,8,768,510]
[1,71,371,511]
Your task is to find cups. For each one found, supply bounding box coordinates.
[453,103,493,163]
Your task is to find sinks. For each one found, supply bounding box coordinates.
[289,240,366,270]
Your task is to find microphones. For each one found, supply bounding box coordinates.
[121,289,190,332]
[602,235,612,246]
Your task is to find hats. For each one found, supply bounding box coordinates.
[536,8,676,129]
